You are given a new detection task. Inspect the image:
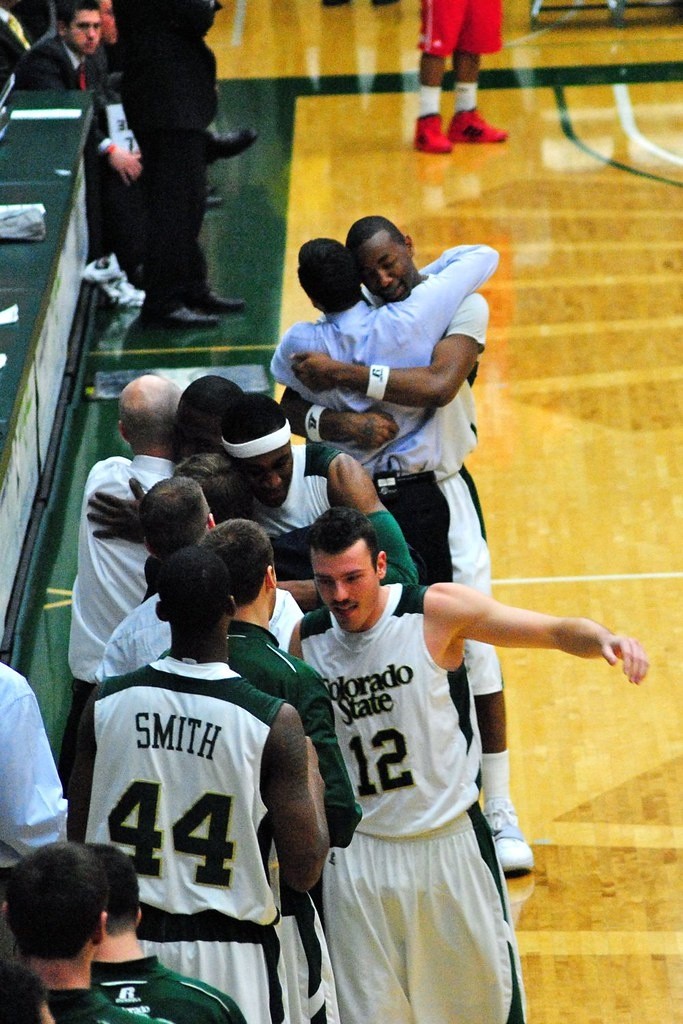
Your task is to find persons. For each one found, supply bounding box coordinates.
[200,517,363,1024]
[187,0,259,208]
[145,450,256,598]
[58,374,184,800]
[72,544,330,1024]
[138,476,214,555]
[270,215,535,876]
[223,390,418,612]
[321,0,402,8]
[0,958,56,1024]
[32,0,123,102]
[87,374,245,544]
[413,0,509,153]
[0,660,68,868]
[0,839,176,1024]
[0,0,37,91]
[84,842,248,1024]
[14,0,147,308]
[289,506,649,1024]
[110,0,247,327]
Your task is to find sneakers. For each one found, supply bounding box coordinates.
[448,109,507,143]
[415,113,453,154]
[484,797,534,873]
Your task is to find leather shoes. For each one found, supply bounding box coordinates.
[148,304,221,331]
[196,290,244,314]
[204,125,255,160]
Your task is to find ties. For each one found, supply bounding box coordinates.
[79,63,86,91]
[8,15,31,50]
